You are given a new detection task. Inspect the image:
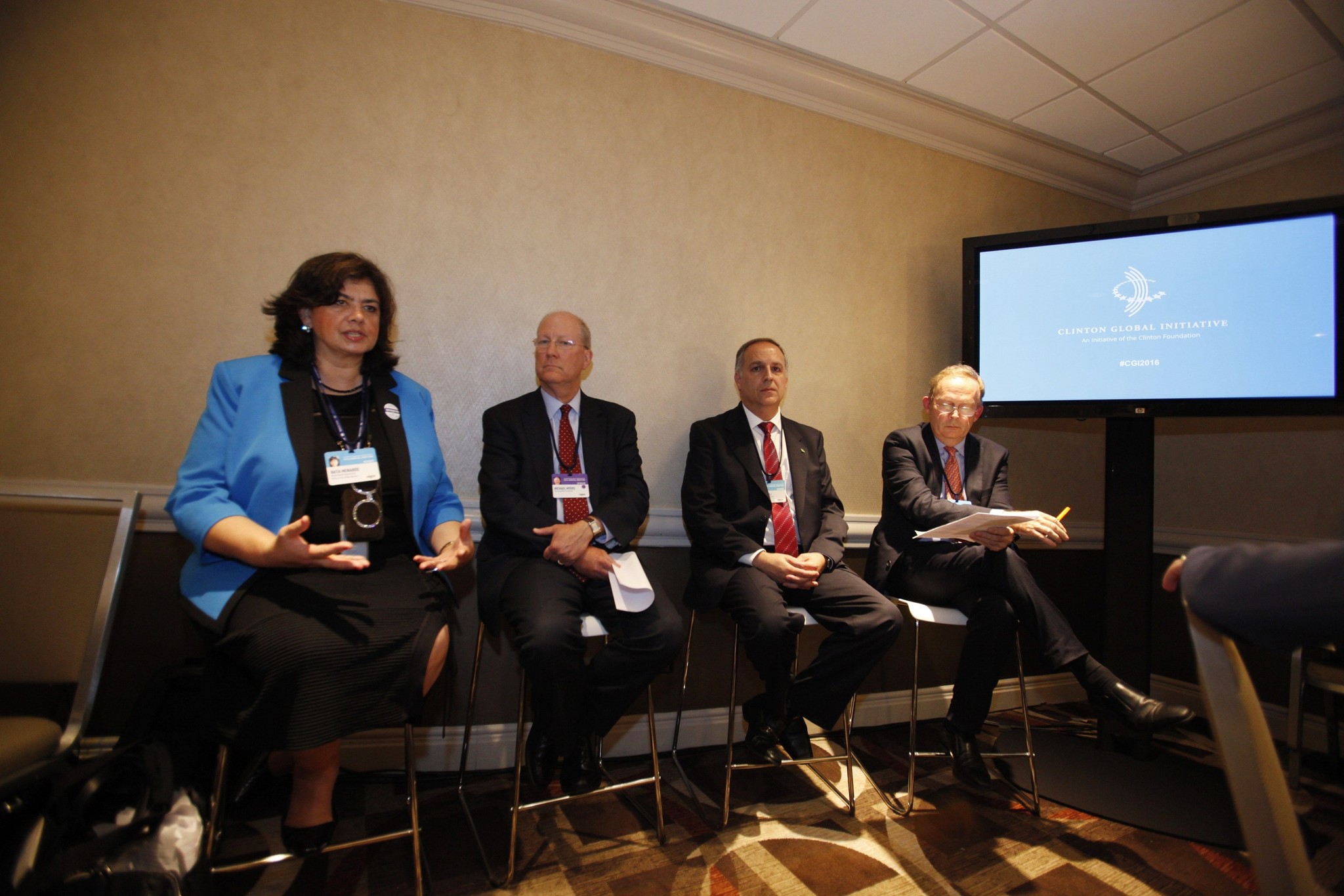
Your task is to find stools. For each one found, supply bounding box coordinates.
[884,589,1043,820]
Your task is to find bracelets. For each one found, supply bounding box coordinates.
[438,540,458,555]
[824,555,830,570]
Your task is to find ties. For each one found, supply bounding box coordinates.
[943,445,971,545]
[757,422,799,559]
[559,404,590,583]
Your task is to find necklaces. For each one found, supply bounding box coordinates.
[322,377,363,393]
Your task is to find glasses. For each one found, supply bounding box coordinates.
[532,338,589,350]
[930,402,978,417]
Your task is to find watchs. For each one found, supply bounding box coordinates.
[582,517,601,537]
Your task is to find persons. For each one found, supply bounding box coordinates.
[1163,537,1344,642]
[474,310,684,793]
[167,252,476,860]
[861,364,1196,789]
[680,337,902,768]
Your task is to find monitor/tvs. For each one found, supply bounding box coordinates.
[963,190,1343,419]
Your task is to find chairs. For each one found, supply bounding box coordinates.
[670,542,864,836]
[205,715,428,896]
[452,492,671,888]
[1,480,146,896]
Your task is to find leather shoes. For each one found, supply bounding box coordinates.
[742,692,787,768]
[230,748,272,802]
[766,672,814,759]
[524,687,558,791]
[559,725,601,796]
[938,719,992,792]
[280,776,339,857]
[1086,679,1196,731]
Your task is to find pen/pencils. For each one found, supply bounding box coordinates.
[1045,506,1071,539]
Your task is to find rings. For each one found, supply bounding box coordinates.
[557,559,564,565]
[439,559,443,563]
